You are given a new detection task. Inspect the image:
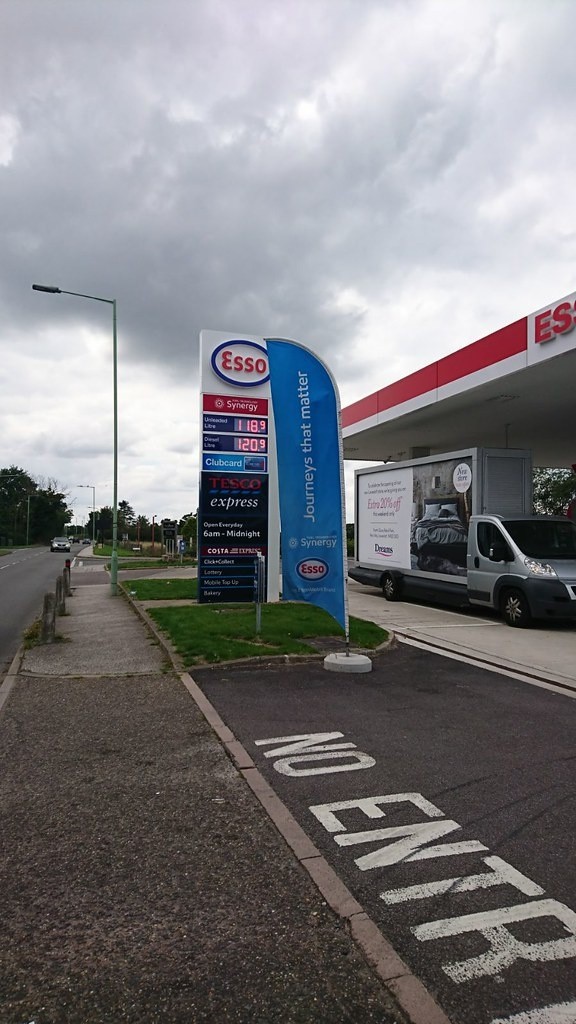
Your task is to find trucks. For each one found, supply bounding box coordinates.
[347,447,576,629]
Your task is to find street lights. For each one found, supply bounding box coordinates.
[88,506,100,542]
[76,485,95,548]
[27,495,46,545]
[152,515,157,547]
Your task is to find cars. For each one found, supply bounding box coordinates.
[82,539,91,544]
[68,535,74,541]
[32,284,119,598]
[51,537,72,552]
[73,537,80,544]
